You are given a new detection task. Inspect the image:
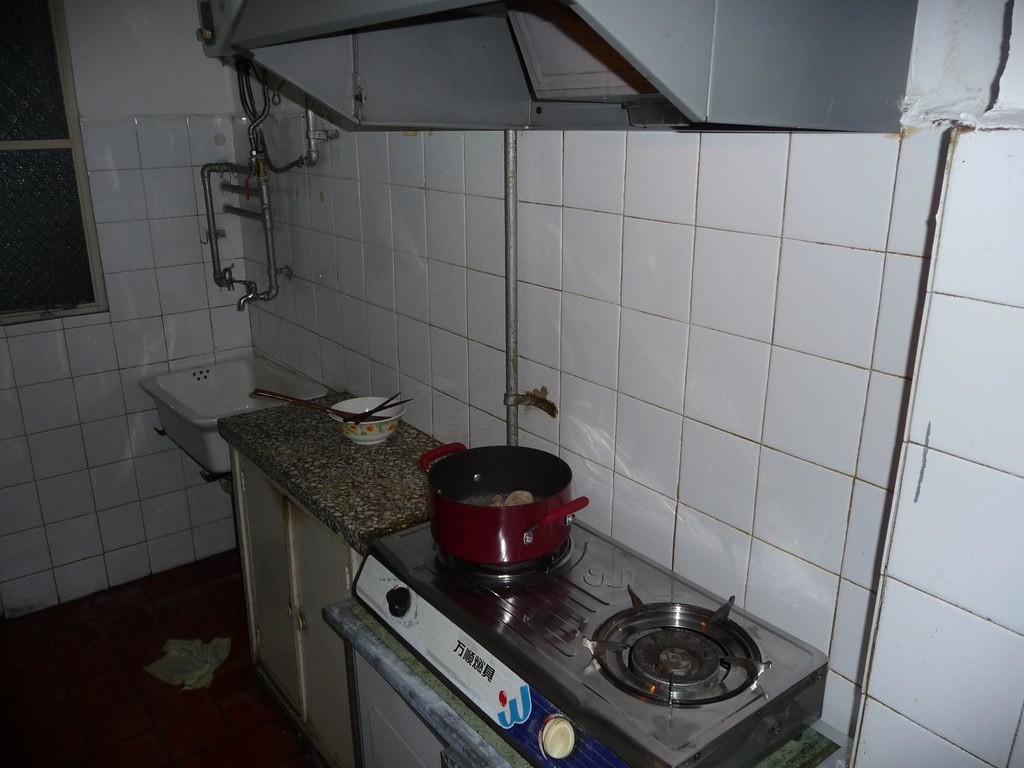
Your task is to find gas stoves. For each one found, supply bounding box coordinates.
[348,514,828,768]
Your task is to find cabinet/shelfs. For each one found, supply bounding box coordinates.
[352,647,456,768]
[229,442,365,767]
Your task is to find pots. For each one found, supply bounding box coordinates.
[419,442,589,565]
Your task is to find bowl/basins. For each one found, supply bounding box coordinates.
[327,396,406,446]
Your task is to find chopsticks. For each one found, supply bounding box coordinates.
[343,392,413,425]
